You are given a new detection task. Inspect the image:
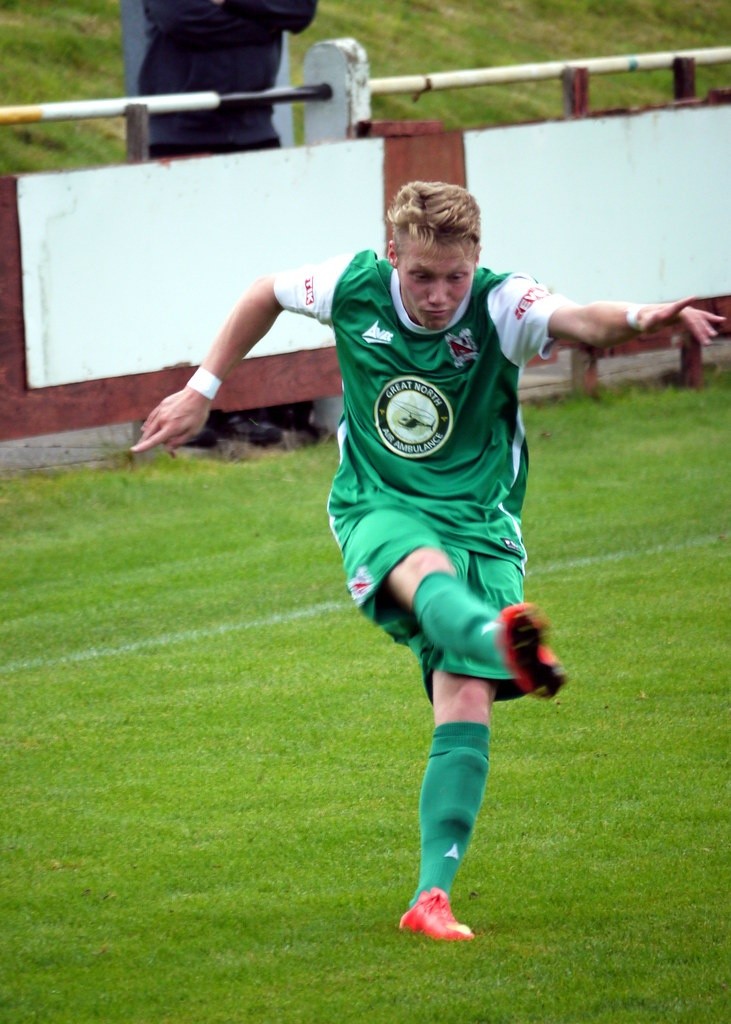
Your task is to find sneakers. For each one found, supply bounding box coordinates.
[398,890,477,941]
[492,601,567,702]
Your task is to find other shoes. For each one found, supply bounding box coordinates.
[205,410,282,445]
[179,423,218,448]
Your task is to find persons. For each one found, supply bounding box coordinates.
[130,181,728,938]
[121,0,320,448]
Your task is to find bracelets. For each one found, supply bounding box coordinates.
[627,305,644,331]
[186,367,222,400]
